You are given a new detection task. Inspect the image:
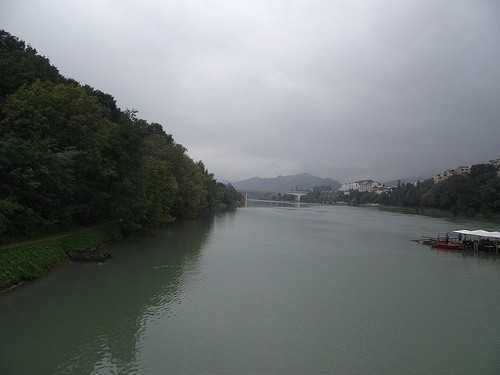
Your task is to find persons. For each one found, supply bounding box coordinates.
[462,236,500,246]
[458,233,462,241]
[446,232,449,243]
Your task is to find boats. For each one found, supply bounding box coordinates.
[432,241,464,248]
[67,246,111,261]
